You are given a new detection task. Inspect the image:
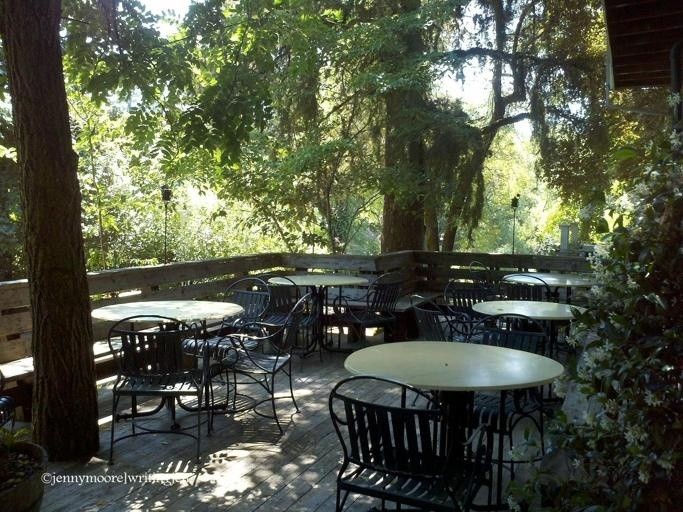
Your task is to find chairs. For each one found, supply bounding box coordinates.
[106,260,551,512]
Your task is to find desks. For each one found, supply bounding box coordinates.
[90,299,246,431]
[344,273,606,509]
